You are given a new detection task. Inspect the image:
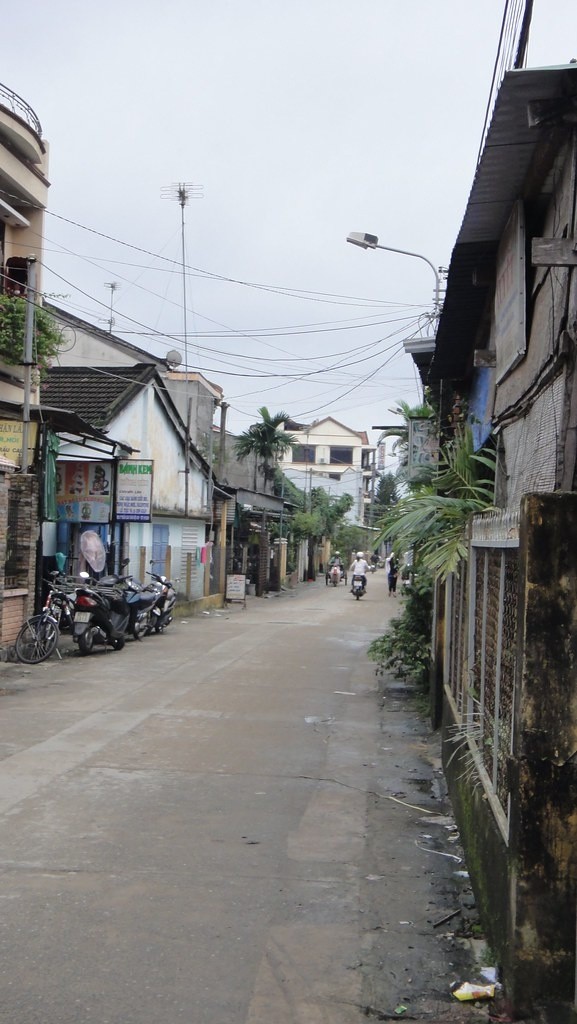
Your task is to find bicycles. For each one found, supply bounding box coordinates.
[15,571,77,663]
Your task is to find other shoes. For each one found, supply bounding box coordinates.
[393,593,396,597]
[351,589,353,592]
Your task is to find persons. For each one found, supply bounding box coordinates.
[386,552,400,597]
[350,552,371,589]
[329,551,344,573]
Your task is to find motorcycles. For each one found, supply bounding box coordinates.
[325,563,347,587]
[370,562,377,574]
[352,575,365,600]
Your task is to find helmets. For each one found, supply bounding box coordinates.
[335,551,340,555]
[357,552,364,558]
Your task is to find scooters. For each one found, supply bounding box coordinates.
[73,556,133,655]
[115,560,181,641]
